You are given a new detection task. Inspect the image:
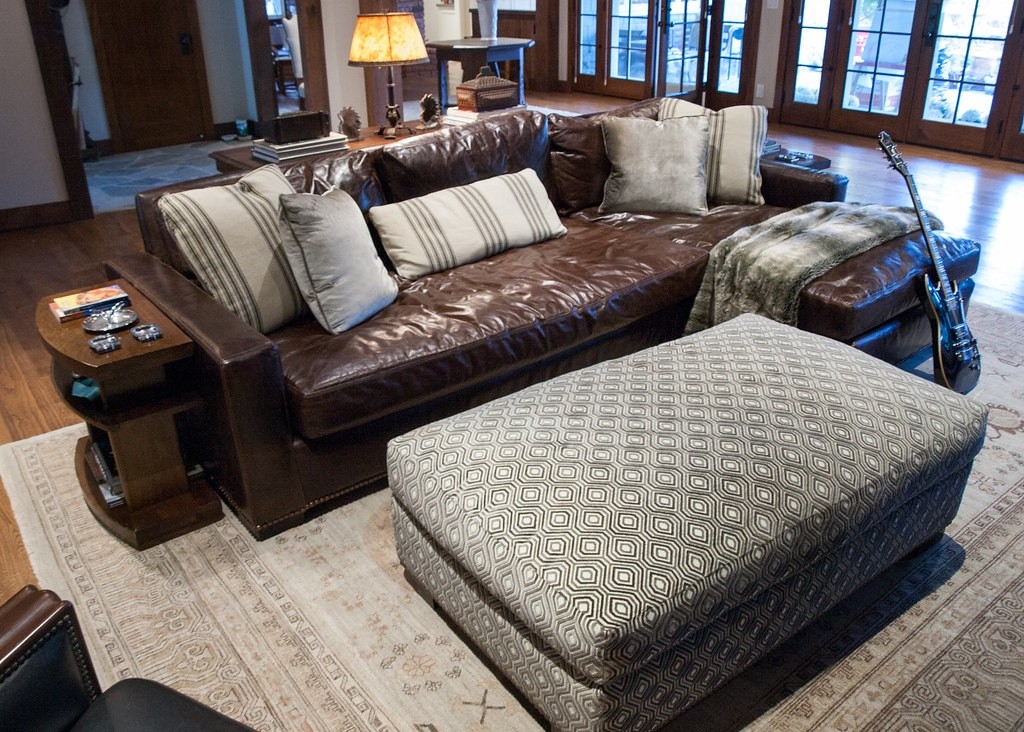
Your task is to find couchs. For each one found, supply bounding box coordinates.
[104,99,857,544]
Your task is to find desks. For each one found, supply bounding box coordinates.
[207,110,512,175]
[423,37,536,107]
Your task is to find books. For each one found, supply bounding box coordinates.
[47,283,131,324]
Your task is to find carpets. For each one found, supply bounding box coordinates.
[0,297,1024,732]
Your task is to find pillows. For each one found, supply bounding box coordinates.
[370,168,570,279]
[599,114,709,218]
[273,188,399,335]
[657,100,772,203]
[156,164,304,334]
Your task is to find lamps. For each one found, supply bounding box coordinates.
[345,13,431,139]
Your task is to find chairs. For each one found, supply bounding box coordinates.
[0,584,266,732]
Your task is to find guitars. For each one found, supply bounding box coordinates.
[875,129,983,396]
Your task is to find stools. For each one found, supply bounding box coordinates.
[732,229,981,371]
[389,313,985,731]
